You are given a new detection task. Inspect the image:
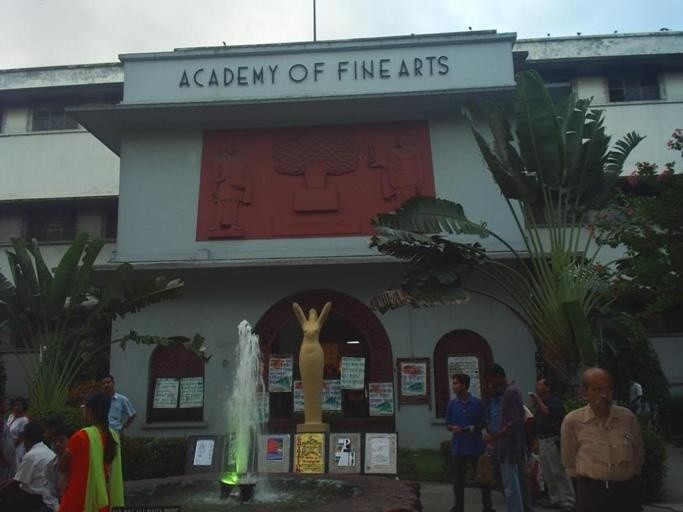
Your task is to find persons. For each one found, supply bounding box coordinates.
[0,374,137,512]
[445,363,646,512]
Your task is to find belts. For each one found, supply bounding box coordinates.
[578,474,637,490]
